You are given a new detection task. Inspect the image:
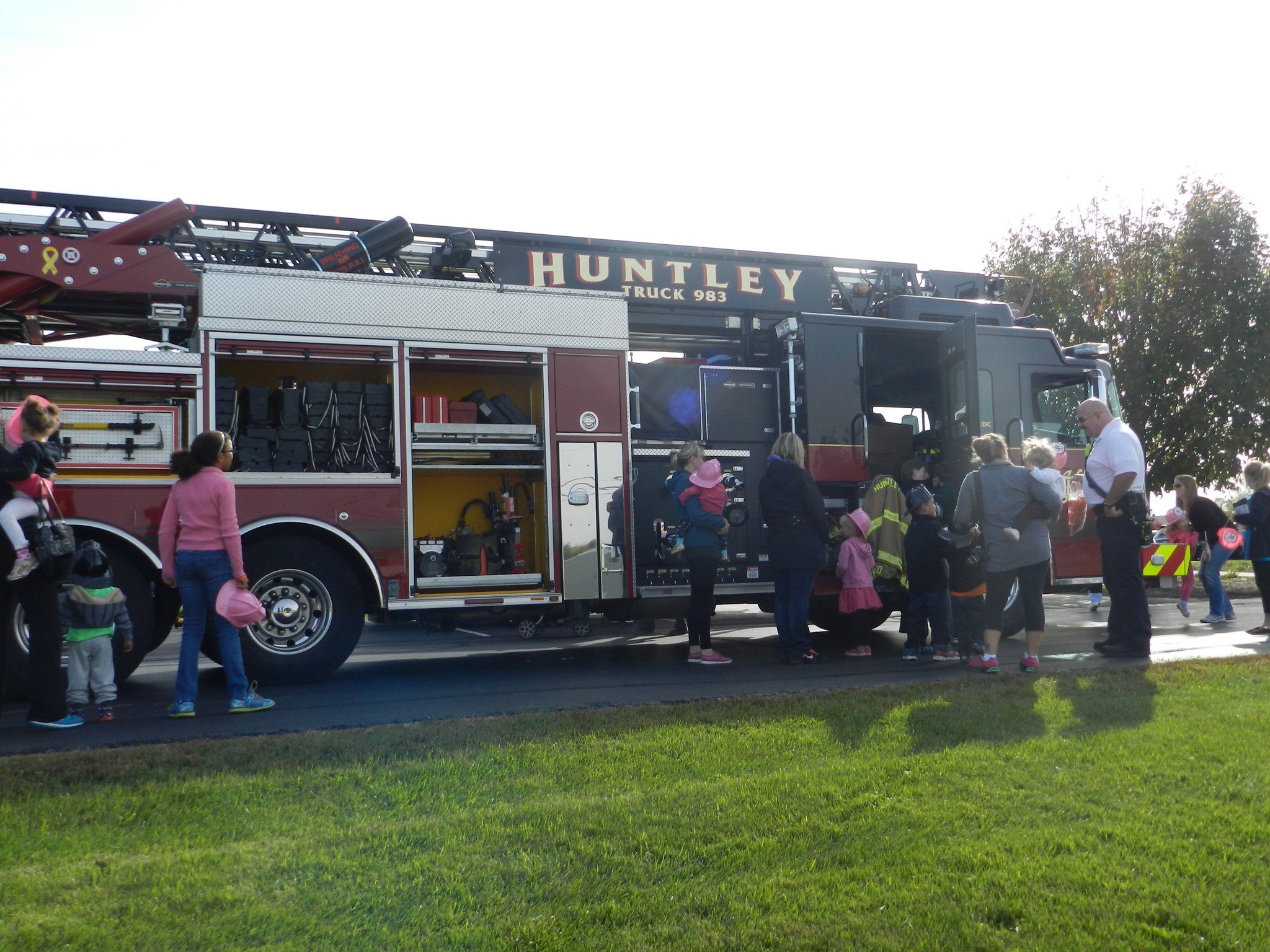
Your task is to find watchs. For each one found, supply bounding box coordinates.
[1103,500,1115,509]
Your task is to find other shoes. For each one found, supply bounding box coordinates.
[1177,601,1270,635]
[1093,639,1117,652]
[1090,601,1099,611]
[99,712,114,721]
[1102,644,1149,658]
[671,544,686,555]
[721,553,730,564]
[1003,526,1020,541]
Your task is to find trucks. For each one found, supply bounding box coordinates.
[0,190,1144,687]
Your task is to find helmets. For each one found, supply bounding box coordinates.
[903,483,935,519]
[73,539,110,578]
[216,580,266,627]
[846,507,871,543]
[688,458,723,488]
[1217,527,1244,550]
[1162,509,1185,526]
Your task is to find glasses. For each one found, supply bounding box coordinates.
[222,447,237,455]
[1079,410,1105,422]
[1173,483,1185,488]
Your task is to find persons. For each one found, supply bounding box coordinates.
[758,432,834,664]
[1087,583,1103,612]
[0,395,76,728]
[60,546,133,721]
[1173,475,1238,623]
[664,442,732,664]
[158,430,276,718]
[1069,398,1152,659]
[1165,507,1199,618]
[1231,460,1270,635]
[836,507,885,656]
[897,431,1067,673]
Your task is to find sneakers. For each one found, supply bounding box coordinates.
[165,700,196,717]
[28,714,84,729]
[687,652,702,663]
[700,650,732,664]
[780,636,1039,674]
[7,556,39,581]
[229,680,276,713]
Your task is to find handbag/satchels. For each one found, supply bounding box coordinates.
[34,479,75,561]
[1202,532,1211,562]
[451,498,514,576]
[1141,520,1154,546]
